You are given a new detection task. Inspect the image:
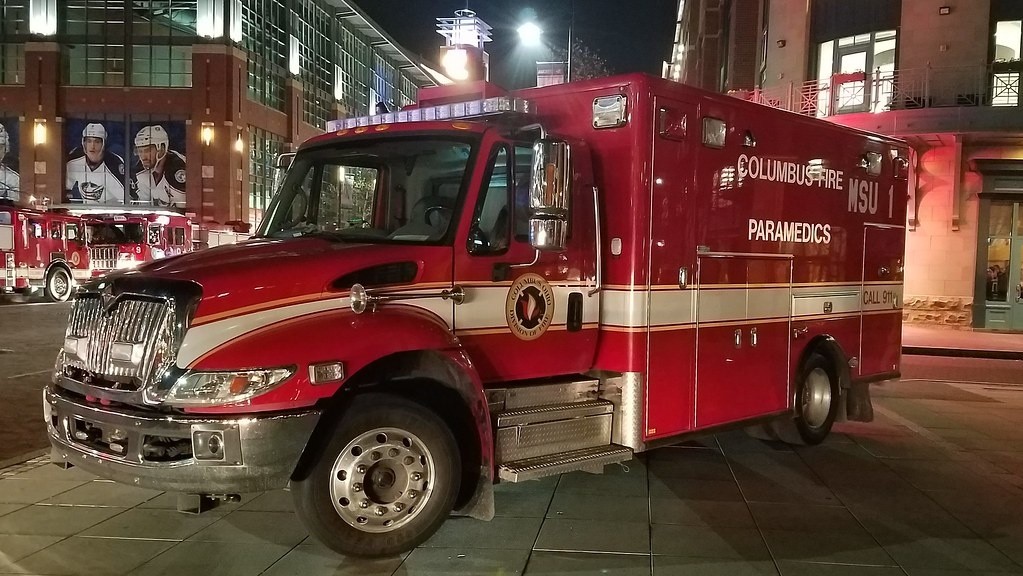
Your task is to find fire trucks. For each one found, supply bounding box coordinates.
[0,204,195,304]
[40,71,918,561]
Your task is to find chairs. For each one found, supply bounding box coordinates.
[491,175,529,251]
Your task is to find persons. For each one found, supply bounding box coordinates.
[0,124,20,202]
[133,125,186,208]
[63,122,139,206]
[989,265,1006,301]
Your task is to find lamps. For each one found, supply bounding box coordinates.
[776,39,786,48]
[938,7,952,15]
